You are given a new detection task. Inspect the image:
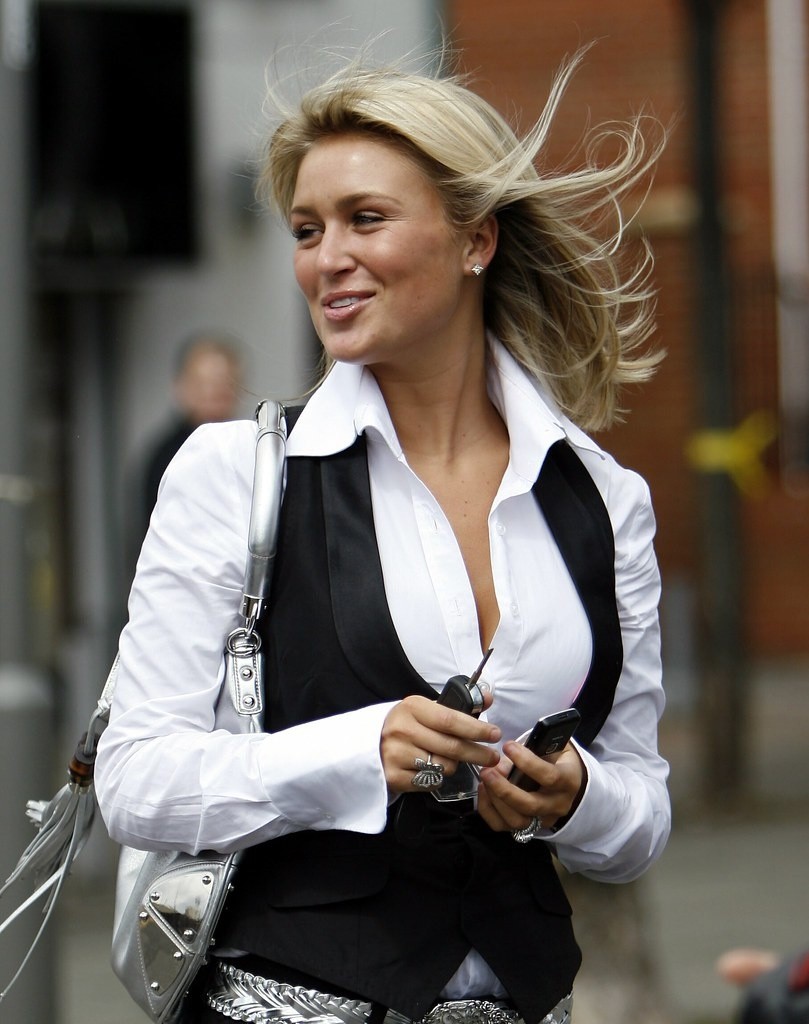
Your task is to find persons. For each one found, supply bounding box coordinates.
[93,39,672,1024]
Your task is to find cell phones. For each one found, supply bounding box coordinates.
[507,709,580,794]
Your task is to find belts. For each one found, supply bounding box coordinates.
[206,949,572,1024]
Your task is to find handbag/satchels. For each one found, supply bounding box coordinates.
[0,400,287,1024]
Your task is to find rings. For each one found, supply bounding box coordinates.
[513,815,542,844]
[411,753,444,787]
